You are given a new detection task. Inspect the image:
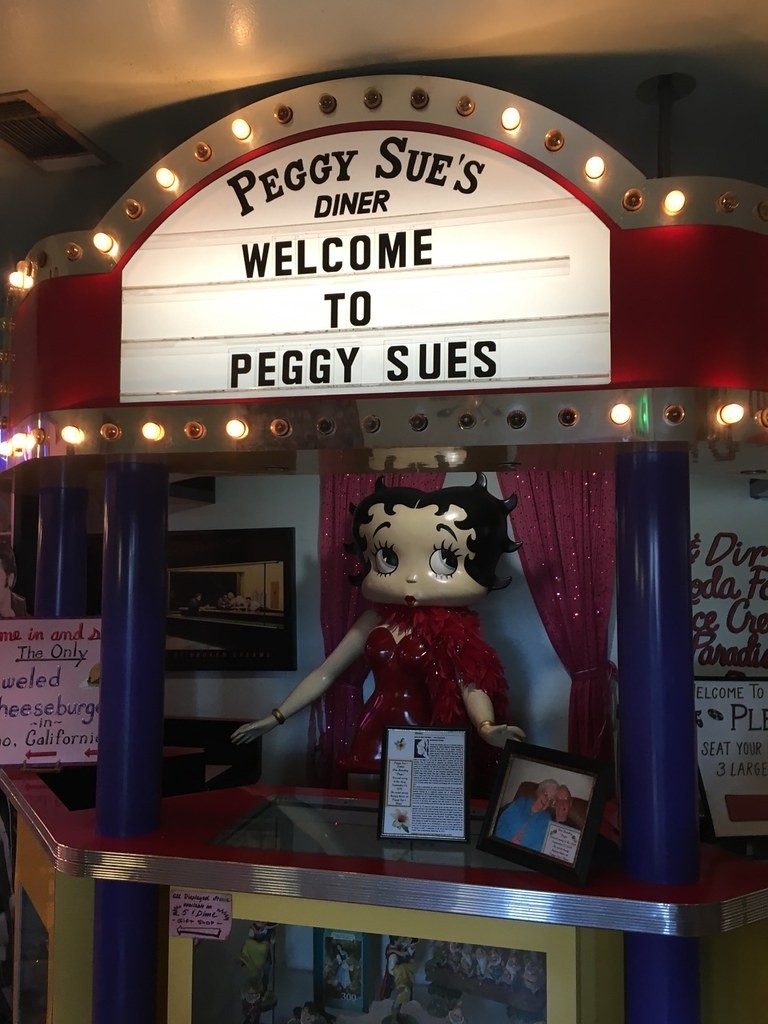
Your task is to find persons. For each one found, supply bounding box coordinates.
[553,784,581,831]
[496,779,558,853]
[379,936,548,1023]
[231,470,530,782]
[240,921,277,1003]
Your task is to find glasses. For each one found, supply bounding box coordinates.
[543,790,553,803]
[554,796,570,802]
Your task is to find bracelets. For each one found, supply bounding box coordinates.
[272,708,286,725]
[478,721,495,735]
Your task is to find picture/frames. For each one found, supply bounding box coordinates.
[477,738,615,888]
